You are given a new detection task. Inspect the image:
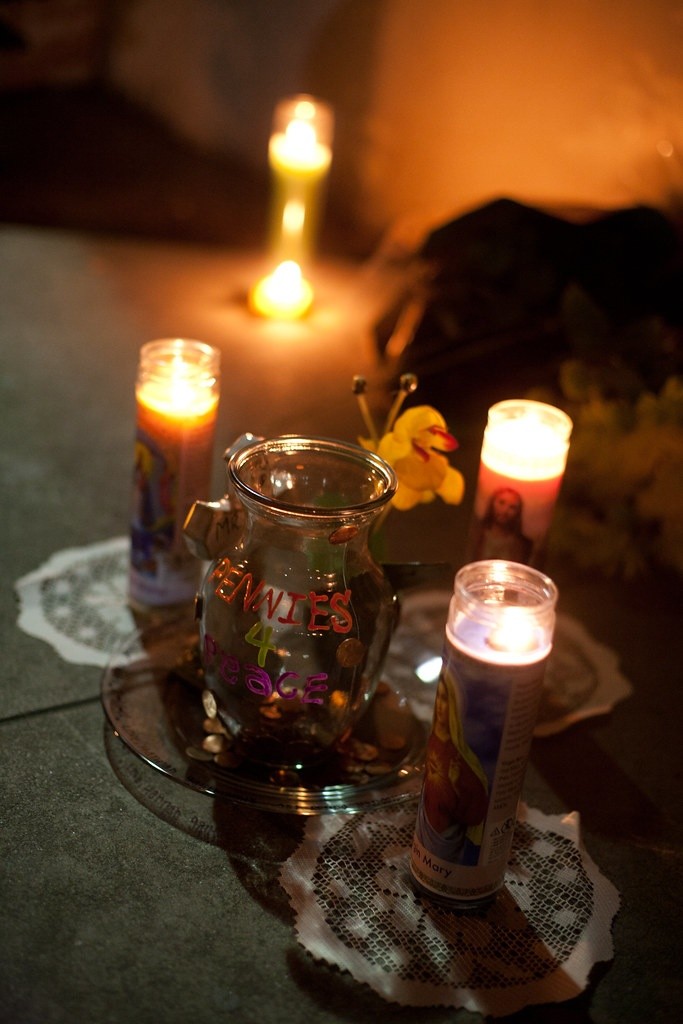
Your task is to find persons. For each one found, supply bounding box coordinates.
[0,0,683,391]
[467,487,534,566]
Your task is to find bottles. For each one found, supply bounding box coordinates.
[200,433,401,780]
